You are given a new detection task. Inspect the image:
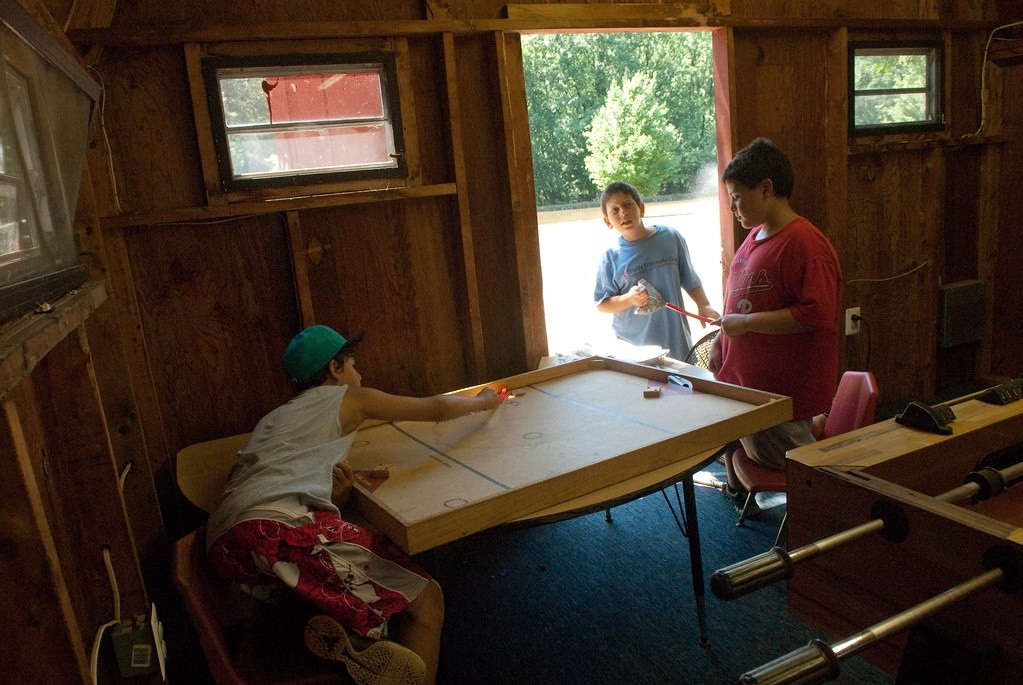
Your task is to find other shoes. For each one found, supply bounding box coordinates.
[304,614,428,685]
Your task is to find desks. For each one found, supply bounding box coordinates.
[501,443,732,648]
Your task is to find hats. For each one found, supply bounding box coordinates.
[279,325,366,386]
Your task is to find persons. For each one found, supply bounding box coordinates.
[706,138,843,470]
[207,323,499,685]
[593,181,737,366]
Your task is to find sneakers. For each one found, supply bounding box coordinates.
[721,481,761,518]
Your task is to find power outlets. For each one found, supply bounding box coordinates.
[845,307,861,335]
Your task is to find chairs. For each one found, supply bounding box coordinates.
[172,525,344,685]
[732,372,879,545]
